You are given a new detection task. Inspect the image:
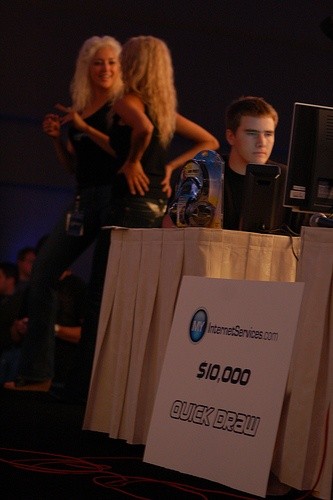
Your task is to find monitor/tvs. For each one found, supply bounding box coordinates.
[239,164,310,237]
[283,102,333,218]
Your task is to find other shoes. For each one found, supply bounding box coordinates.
[5,378,53,391]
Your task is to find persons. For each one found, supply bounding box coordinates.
[0,35,219,393]
[219,96,311,234]
